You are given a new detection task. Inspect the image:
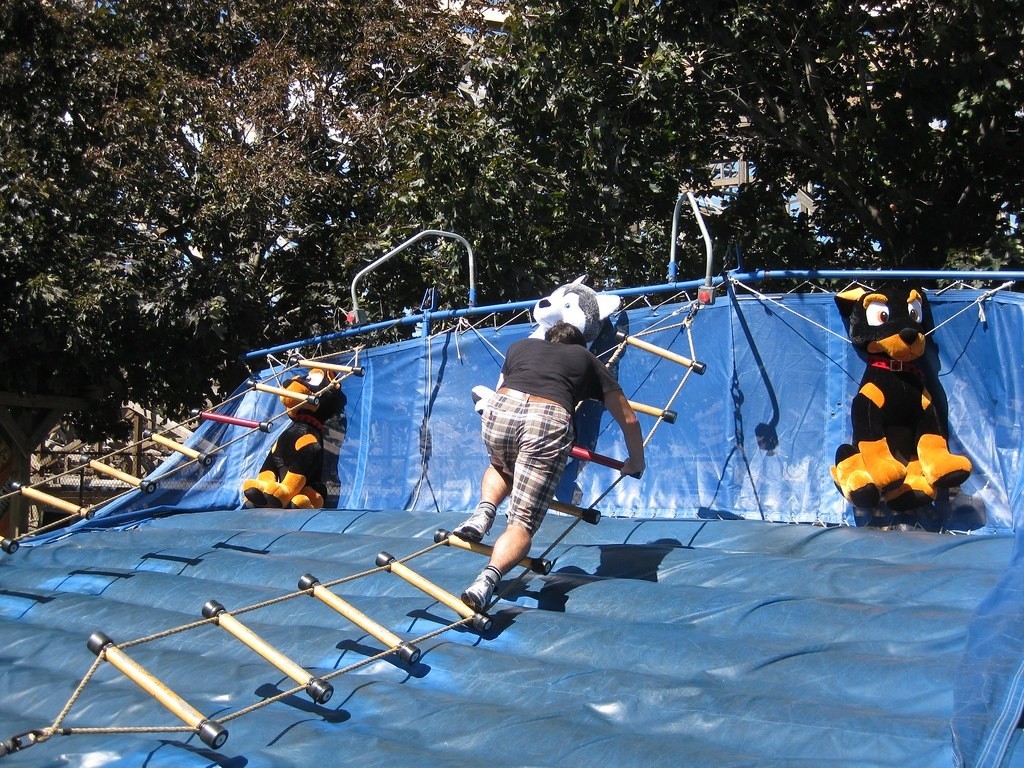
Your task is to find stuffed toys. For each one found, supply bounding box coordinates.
[829,278,973,511]
[470,273,621,414]
[244,368,346,509]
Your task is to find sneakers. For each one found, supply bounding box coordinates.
[452,511,495,544]
[460,576,495,614]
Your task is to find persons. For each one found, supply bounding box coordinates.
[454,323,646,627]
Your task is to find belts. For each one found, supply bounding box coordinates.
[496,386,564,407]
